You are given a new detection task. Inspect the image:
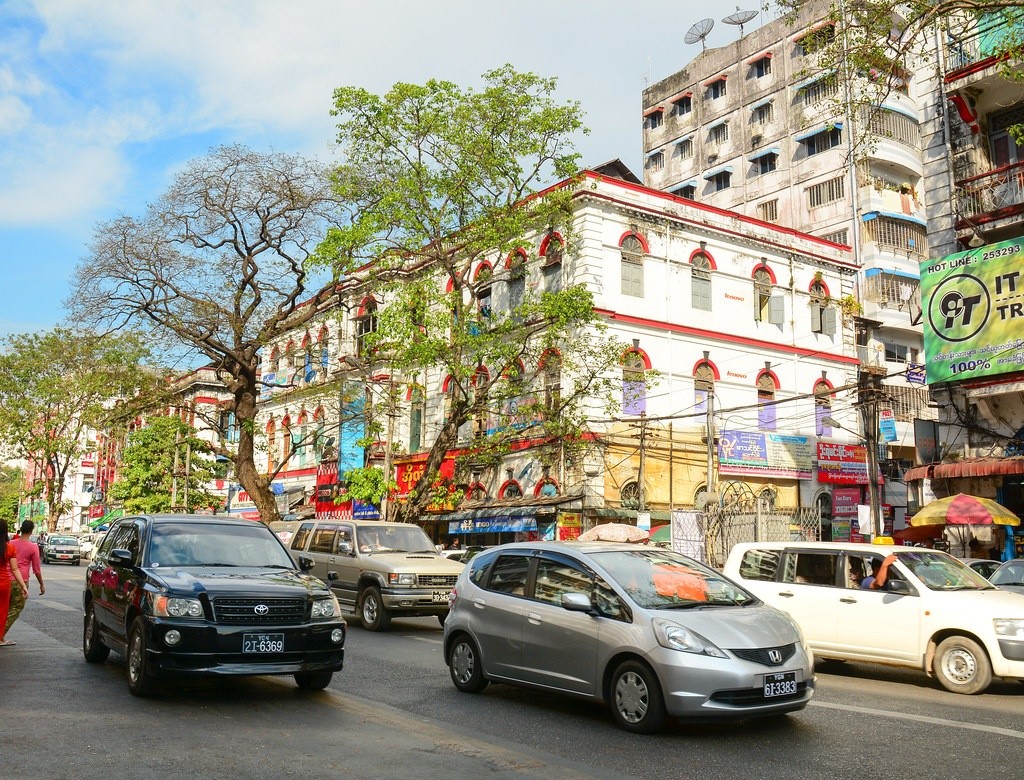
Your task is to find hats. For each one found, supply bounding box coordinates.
[39,531,45,535]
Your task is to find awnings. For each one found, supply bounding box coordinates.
[419,507,556,532]
[903,456,1024,481]
[85,508,123,528]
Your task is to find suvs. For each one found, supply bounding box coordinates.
[722,534,1024,696]
[441,540,819,735]
[83,513,347,698]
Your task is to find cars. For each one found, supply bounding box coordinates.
[912,557,1016,587]
[29,530,108,566]
[440,543,501,565]
[985,558,1024,596]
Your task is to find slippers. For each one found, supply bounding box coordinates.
[1,640,17,645]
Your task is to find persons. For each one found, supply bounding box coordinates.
[0,520,45,646]
[450,538,460,552]
[12,530,20,539]
[38,530,46,551]
[862,553,897,589]
[439,539,448,550]
[922,537,934,549]
[86,565,139,617]
[338,532,355,554]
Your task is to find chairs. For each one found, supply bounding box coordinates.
[849,558,865,589]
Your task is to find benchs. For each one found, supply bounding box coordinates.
[797,573,832,585]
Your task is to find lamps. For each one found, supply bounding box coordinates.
[968,228,985,247]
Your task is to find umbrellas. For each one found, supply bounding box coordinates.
[577,523,650,542]
[909,492,1020,557]
[651,525,672,543]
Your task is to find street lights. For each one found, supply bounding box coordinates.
[820,416,882,536]
[344,355,398,521]
[215,456,233,517]
[1,472,24,530]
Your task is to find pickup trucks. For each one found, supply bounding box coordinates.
[269,518,469,633]
